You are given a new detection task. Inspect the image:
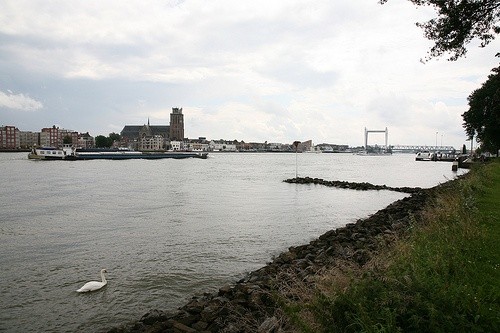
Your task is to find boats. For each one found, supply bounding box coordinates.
[30,142,208,159]
[413,150,469,161]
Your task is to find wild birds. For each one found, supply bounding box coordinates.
[75,268,108,293]
